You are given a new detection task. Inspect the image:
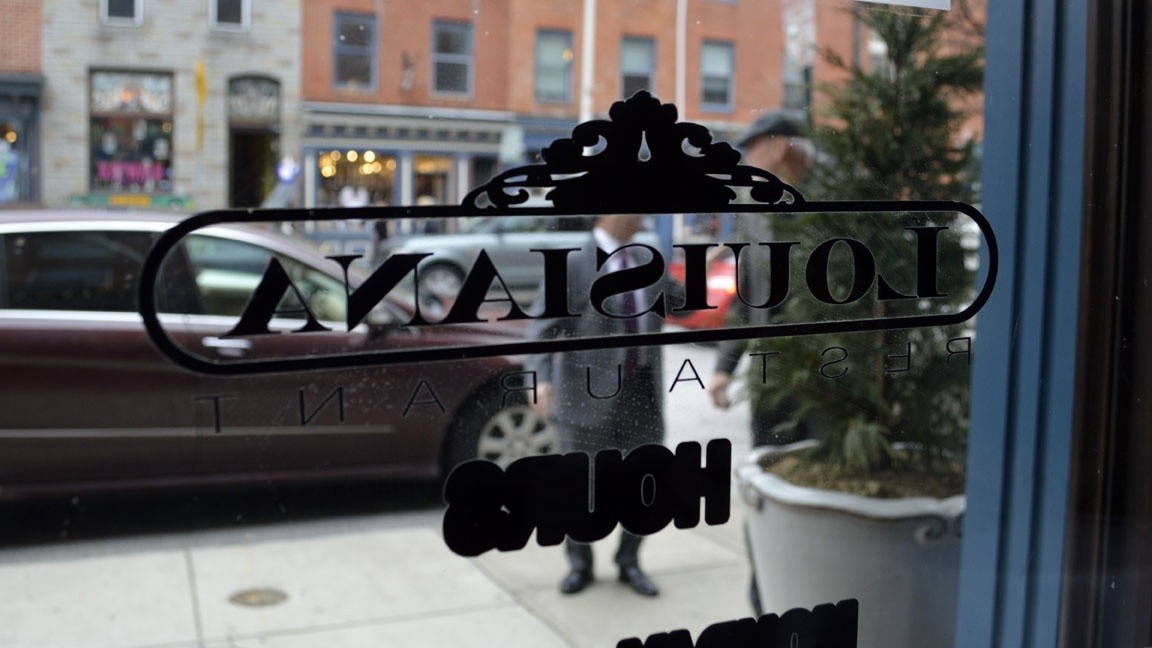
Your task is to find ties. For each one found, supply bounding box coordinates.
[619,246,649,369]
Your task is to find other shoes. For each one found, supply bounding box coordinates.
[617,566,658,596]
[560,562,595,593]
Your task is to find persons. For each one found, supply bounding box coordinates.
[370,200,397,241]
[523,215,729,598]
[705,112,845,446]
[412,194,440,234]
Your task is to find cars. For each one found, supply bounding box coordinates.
[660,245,746,329]
[373,194,595,313]
[0,205,565,517]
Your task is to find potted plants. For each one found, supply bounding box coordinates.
[727,0,987,648]
